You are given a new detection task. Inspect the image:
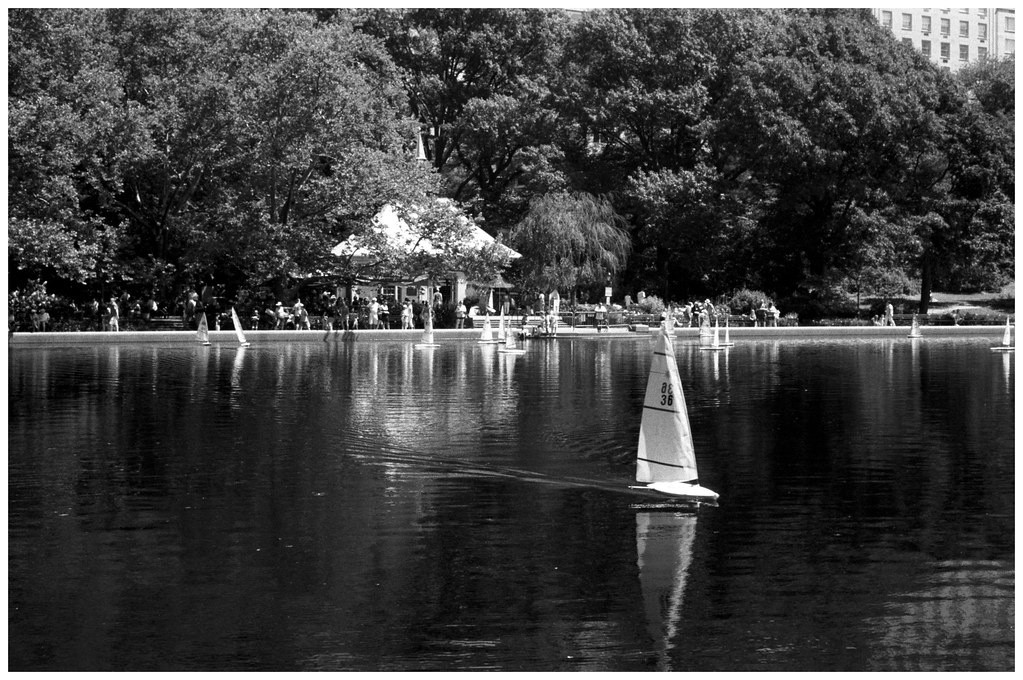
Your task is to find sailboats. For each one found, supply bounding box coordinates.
[720,316,734,346]
[989,315,1015,350]
[230,306,251,349]
[627,307,720,503]
[992,350,1014,393]
[907,312,923,337]
[194,312,212,346]
[414,305,442,347]
[495,306,507,343]
[629,503,701,670]
[476,312,495,344]
[696,317,725,350]
[496,317,527,354]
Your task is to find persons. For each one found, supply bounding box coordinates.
[595,302,607,333]
[518,308,557,337]
[290,299,311,330]
[742,298,778,327]
[68,298,99,328]
[124,292,159,325]
[421,301,435,329]
[692,298,718,327]
[105,297,120,331]
[251,310,259,330]
[274,301,285,329]
[365,297,380,330]
[455,300,467,329]
[951,309,964,326]
[320,290,370,329]
[871,301,897,326]
[637,289,646,304]
[188,292,202,330]
[378,300,391,330]
[400,298,417,330]
[625,291,634,312]
[31,307,51,332]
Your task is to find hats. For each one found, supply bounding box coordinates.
[372,297,377,302]
[276,302,282,306]
